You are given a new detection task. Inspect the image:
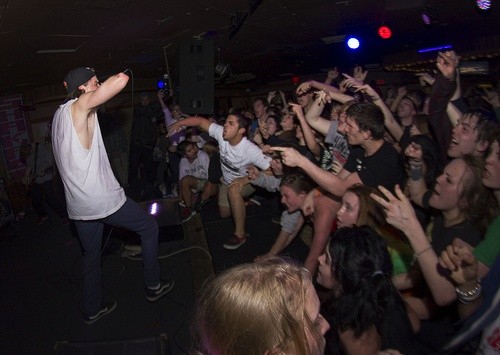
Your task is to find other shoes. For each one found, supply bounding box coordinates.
[181,207,196,222]
[223,234,247,249]
[247,197,262,206]
[271,216,281,222]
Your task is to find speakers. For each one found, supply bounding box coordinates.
[55,333,170,355]
[178,40,215,116]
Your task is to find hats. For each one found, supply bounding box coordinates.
[62,66,96,104]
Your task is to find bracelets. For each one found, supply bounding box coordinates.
[459,286,481,304]
[417,246,433,256]
[456,280,480,296]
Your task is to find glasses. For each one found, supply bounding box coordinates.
[172,108,181,113]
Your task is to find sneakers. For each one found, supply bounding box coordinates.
[145,278,174,301]
[84,299,118,325]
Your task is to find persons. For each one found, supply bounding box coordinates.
[23,125,71,226]
[201,258,330,355]
[133,95,157,183]
[51,66,174,324]
[316,224,421,355]
[157,50,500,321]
